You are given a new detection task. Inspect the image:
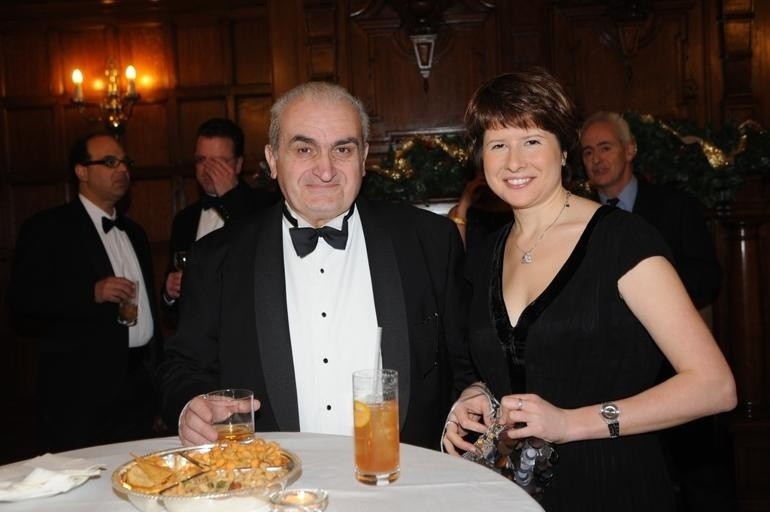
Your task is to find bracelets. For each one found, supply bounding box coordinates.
[448,215,468,225]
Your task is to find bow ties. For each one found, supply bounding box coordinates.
[101,216,126,233]
[289,224,348,257]
[204,196,220,212]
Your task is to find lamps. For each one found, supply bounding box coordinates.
[70,60,140,132]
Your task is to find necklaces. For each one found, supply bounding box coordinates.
[509,189,570,265]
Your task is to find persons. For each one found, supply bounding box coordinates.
[573,110,724,511]
[144,78,466,450]
[446,137,514,264]
[8,129,155,452]
[440,65,741,510]
[159,118,269,318]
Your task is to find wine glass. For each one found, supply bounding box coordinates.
[173,250,187,273]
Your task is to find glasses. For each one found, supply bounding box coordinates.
[84,157,136,170]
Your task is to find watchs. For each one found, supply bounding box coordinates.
[597,397,621,440]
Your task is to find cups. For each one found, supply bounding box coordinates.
[116,280,140,327]
[352,367,402,488]
[204,388,256,446]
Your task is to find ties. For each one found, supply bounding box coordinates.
[608,197,620,206]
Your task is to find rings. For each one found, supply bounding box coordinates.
[517,396,523,411]
